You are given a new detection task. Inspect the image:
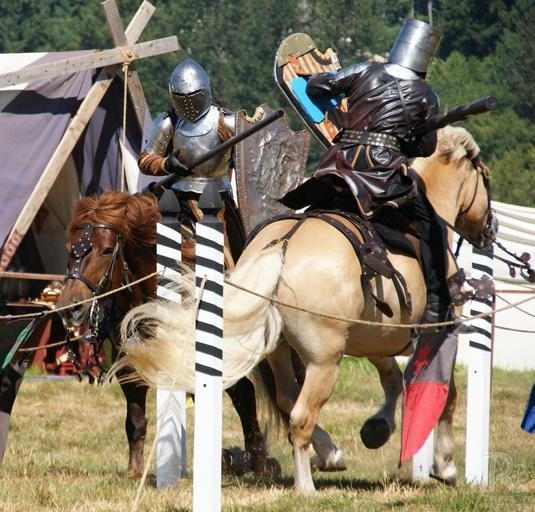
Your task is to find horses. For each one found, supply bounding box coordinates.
[54,188,306,487]
[99,124,501,497]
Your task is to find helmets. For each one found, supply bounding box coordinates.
[169,58,212,122]
[389,18,443,73]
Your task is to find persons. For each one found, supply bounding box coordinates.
[278,19,475,334]
[138,58,247,264]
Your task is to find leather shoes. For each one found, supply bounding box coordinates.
[419,313,473,335]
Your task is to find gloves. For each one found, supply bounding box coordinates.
[165,148,191,176]
